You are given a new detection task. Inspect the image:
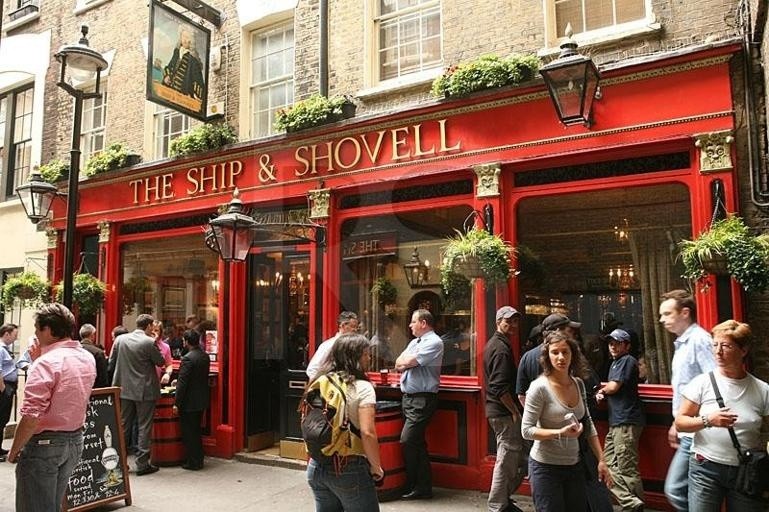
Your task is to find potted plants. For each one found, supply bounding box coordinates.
[31,158,70,182]
[427,54,540,98]
[437,208,521,295]
[167,123,237,158]
[273,92,358,132]
[83,144,142,175]
[677,177,768,296]
[0,270,108,318]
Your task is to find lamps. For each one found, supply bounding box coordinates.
[203,186,326,264]
[13,175,65,227]
[538,22,602,131]
[607,262,639,289]
[403,246,431,290]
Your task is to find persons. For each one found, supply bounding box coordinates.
[659,290,722,512]
[306,310,358,405]
[394,308,444,501]
[7,300,97,511]
[298,333,384,512]
[673,320,768,512]
[359,309,402,370]
[0,304,215,482]
[253,308,309,370]
[161,24,204,97]
[481,305,652,512]
[439,316,470,376]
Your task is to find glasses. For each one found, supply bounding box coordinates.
[712,342,738,349]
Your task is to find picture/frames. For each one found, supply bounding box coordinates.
[145,0,211,122]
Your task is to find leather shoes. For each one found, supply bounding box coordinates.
[0,450,9,455]
[0,457,6,462]
[403,488,435,499]
[182,461,204,470]
[137,465,159,475]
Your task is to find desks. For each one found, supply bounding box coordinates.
[148,384,189,466]
[374,398,410,502]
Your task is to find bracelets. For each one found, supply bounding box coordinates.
[701,415,711,427]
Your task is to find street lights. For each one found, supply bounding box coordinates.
[54,23,108,316]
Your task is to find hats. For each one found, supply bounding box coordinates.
[604,329,630,343]
[542,314,582,331]
[600,317,624,330]
[495,305,521,322]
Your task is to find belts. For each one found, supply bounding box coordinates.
[402,393,438,398]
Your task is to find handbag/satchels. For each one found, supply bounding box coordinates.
[577,416,595,455]
[734,447,769,497]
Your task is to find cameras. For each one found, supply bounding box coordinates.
[564,412,581,432]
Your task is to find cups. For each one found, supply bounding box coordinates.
[390,368,398,388]
[380,369,389,386]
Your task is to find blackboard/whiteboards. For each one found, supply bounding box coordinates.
[63,384,131,512]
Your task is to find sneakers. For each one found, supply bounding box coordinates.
[624,503,644,512]
[501,499,523,512]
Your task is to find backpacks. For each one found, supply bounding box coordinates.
[301,371,368,460]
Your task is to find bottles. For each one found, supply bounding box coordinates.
[103,424,114,447]
